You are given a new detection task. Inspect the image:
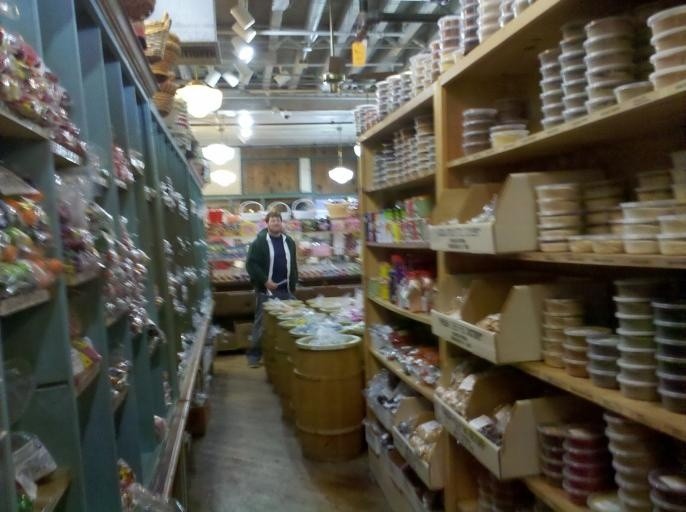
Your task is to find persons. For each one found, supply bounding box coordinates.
[245,213,297,368]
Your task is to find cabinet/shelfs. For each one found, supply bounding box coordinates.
[0,1,213,512]
[357,0,686,512]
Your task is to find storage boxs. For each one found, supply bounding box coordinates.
[213,287,355,352]
[356,172,592,512]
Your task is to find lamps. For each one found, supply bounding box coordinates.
[174,0,361,190]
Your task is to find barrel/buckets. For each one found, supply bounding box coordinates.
[262,296,366,461]
